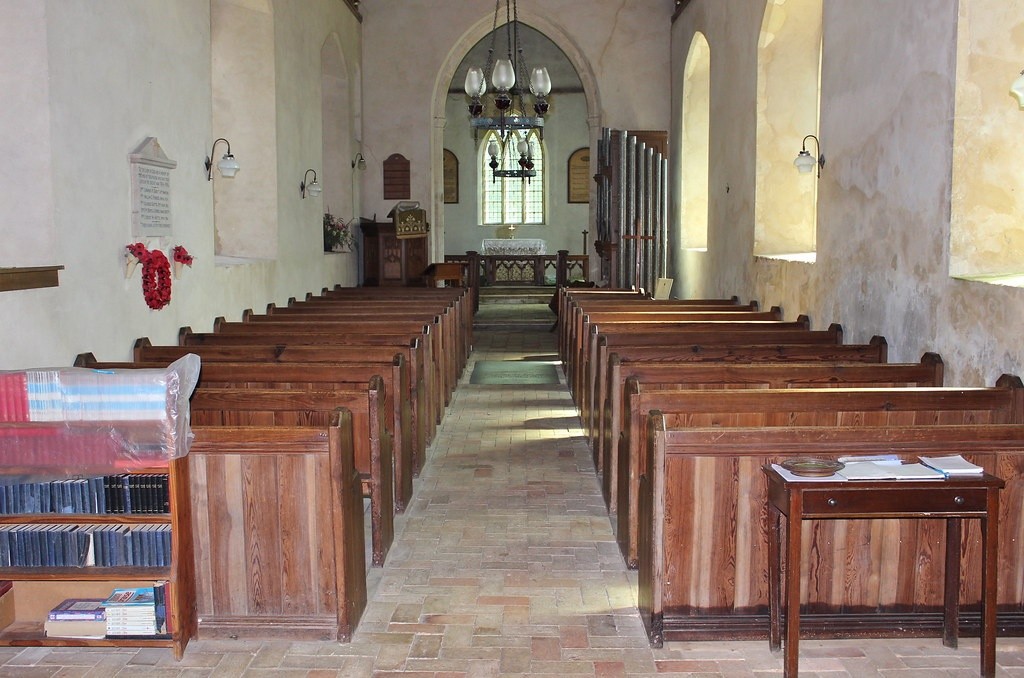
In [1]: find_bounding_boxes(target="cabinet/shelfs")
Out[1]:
[1,364,200,661]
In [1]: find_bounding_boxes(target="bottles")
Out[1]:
[509,225,515,239]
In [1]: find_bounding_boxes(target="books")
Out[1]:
[772,454,983,482]
[0,524,172,568]
[0,423,168,468]
[0,471,170,516]
[0,367,166,423]
[44,581,174,642]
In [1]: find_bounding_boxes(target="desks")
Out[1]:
[760,458,1006,678]
[482,238,547,282]
[420,262,469,287]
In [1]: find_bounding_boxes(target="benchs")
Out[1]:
[73,284,475,645]
[558,285,1024,650]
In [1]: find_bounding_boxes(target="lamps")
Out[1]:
[300,169,322,200]
[794,135,825,178]
[352,152,367,170]
[463,0,552,185]
[205,138,241,182]
